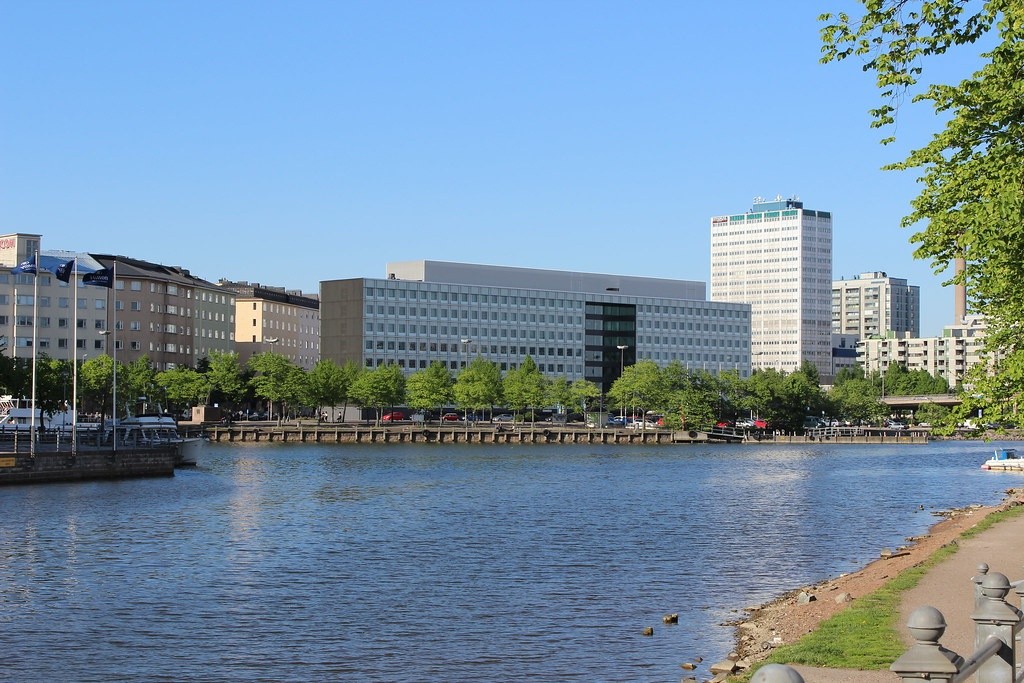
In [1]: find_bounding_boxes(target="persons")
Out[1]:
[321,411,342,422]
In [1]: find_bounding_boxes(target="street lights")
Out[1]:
[81,354,88,414]
[264,337,279,422]
[616,345,630,416]
[99,329,112,357]
[460,338,472,423]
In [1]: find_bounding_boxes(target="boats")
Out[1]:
[981,448,1024,471]
[0,394,102,439]
[103,396,204,465]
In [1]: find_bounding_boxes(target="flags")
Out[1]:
[83,265,115,290]
[10,254,37,277]
[47,259,76,284]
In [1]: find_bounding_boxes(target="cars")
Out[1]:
[442,413,459,422]
[409,410,434,420]
[606,411,911,430]
[382,410,405,421]
[545,411,584,424]
[234,410,294,421]
[461,412,483,421]
[492,413,513,422]
